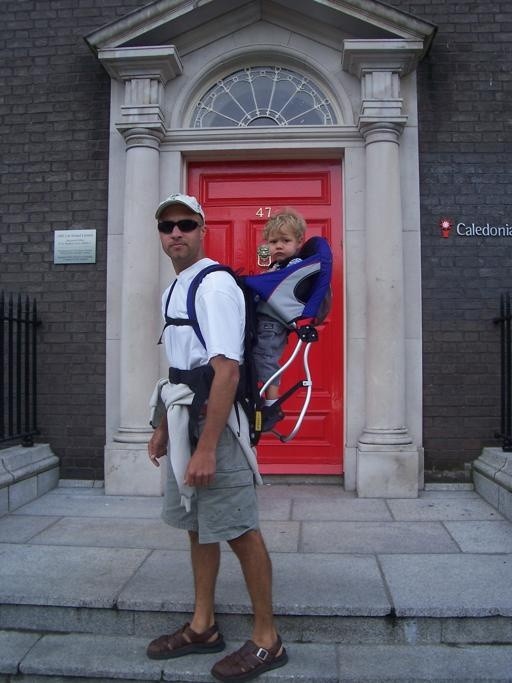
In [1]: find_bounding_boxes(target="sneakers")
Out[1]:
[250,403,286,433]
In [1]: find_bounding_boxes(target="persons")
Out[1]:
[243,206,305,433]
[147,191,289,679]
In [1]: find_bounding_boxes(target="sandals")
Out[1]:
[144,618,227,660]
[209,632,291,681]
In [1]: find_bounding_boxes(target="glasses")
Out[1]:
[156,217,204,233]
